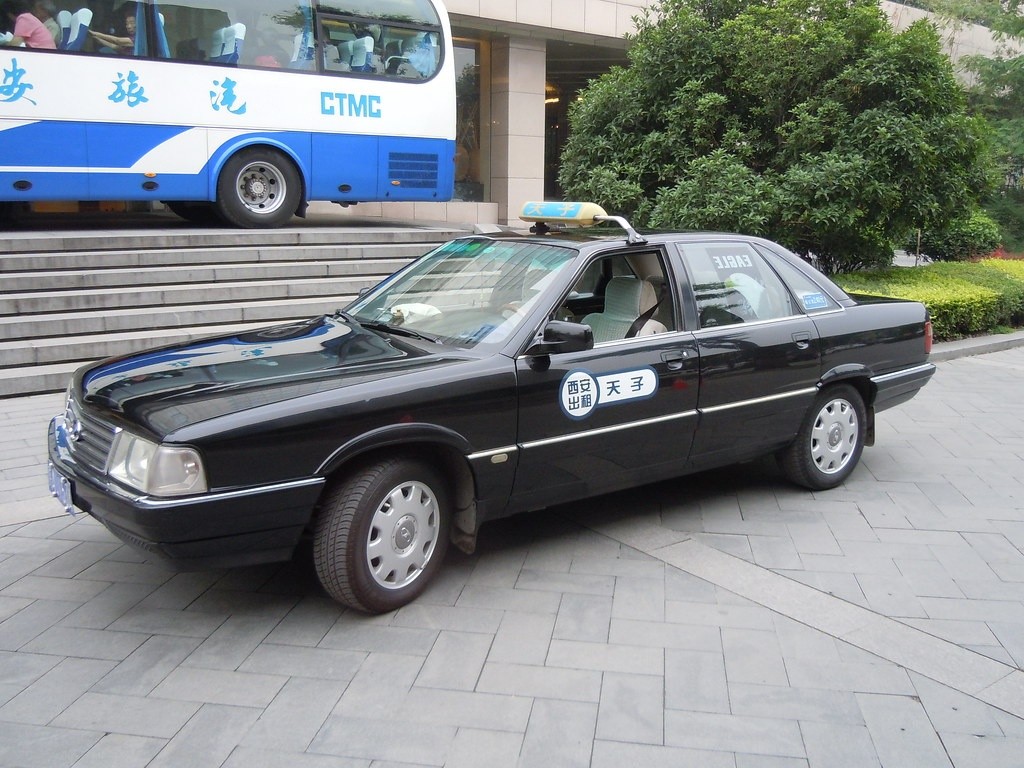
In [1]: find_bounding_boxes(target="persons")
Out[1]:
[88,11,138,56]
[383,42,402,75]
[4,0,58,50]
[246,25,290,68]
[321,24,340,72]
[33,0,62,49]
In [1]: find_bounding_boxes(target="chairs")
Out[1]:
[578,276,668,343]
[0,1,438,78]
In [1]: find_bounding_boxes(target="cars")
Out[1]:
[48,201,937,615]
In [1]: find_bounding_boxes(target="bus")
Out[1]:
[82,321,356,404]
[0,0,457,234]
